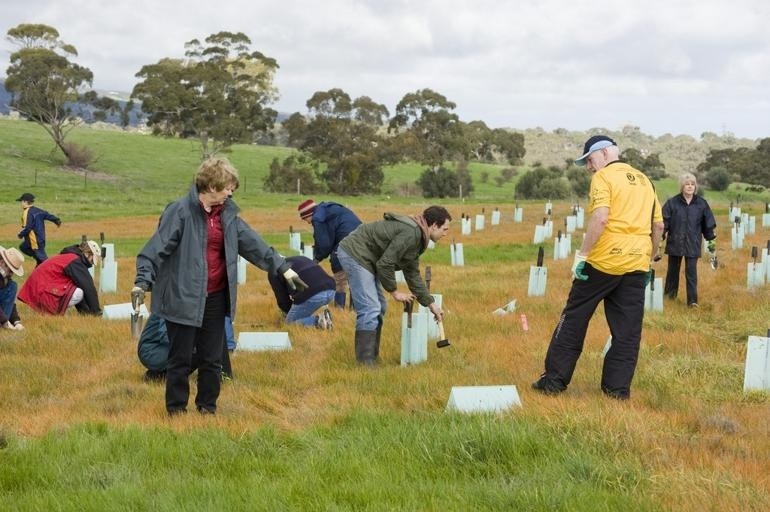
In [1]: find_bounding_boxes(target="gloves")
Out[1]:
[642,265,654,289]
[707,240,716,253]
[130,286,146,310]
[569,249,590,282]
[282,267,310,291]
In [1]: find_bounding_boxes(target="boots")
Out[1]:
[333,291,347,311]
[352,324,387,371]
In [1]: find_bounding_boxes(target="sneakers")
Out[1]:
[319,309,333,331]
[531,371,568,396]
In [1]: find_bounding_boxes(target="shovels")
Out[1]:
[709,242,718,271]
[130,295,143,336]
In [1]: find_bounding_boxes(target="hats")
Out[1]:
[0,244,25,277]
[16,192,35,202]
[679,171,698,195]
[574,134,618,167]
[298,199,317,219]
[87,239,102,266]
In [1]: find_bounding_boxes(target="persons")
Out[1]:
[17,241,103,319]
[268,255,335,332]
[337,205,452,364]
[299,200,362,311]
[0,245,25,330]
[138,309,235,381]
[131,158,309,415]
[661,173,716,307]
[531,135,666,398]
[15,193,61,267]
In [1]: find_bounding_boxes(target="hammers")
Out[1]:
[437,315,450,348]
[654,240,663,262]
